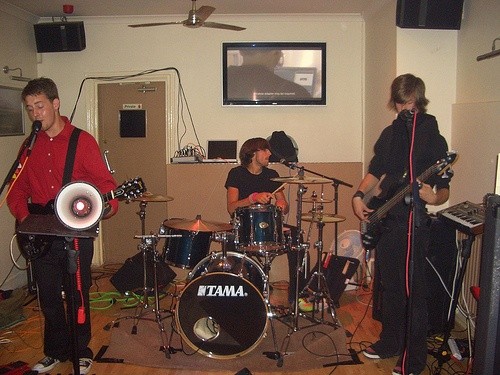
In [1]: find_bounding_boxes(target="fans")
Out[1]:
[128,0,247,31]
[329,229,365,291]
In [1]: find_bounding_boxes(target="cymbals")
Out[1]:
[269,175,333,185]
[296,197,332,203]
[296,212,346,223]
[163,217,234,233]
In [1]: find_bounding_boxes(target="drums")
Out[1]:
[162,227,213,271]
[234,203,283,252]
[222,227,293,256]
[174,250,270,360]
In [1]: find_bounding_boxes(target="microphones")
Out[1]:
[400,108,416,120]
[26,119,43,156]
[280,159,295,171]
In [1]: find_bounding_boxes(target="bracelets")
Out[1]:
[354,191,364,199]
[249,192,257,204]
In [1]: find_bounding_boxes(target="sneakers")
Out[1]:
[72,357,93,374]
[31,355,60,373]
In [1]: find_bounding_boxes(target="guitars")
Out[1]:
[14,175,148,262]
[359,150,458,250]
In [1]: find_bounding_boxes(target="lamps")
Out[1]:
[476,38,500,61]
[3,66,32,82]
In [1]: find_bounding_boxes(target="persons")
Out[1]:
[352,73,454,375]
[227,49,311,98]
[7,78,118,375]
[225,138,312,312]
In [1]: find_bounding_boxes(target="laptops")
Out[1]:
[202,140,237,162]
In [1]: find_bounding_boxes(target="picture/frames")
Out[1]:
[0,85,25,137]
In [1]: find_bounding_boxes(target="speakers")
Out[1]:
[34,21,86,54]
[110,248,177,298]
[395,0,464,30]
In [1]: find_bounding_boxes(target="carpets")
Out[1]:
[92,282,365,371]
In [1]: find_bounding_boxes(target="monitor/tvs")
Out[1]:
[222,42,326,105]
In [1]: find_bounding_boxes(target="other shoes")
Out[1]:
[392,365,409,375]
[363,347,380,358]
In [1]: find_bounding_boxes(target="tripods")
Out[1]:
[269,182,352,367]
[104,203,193,360]
